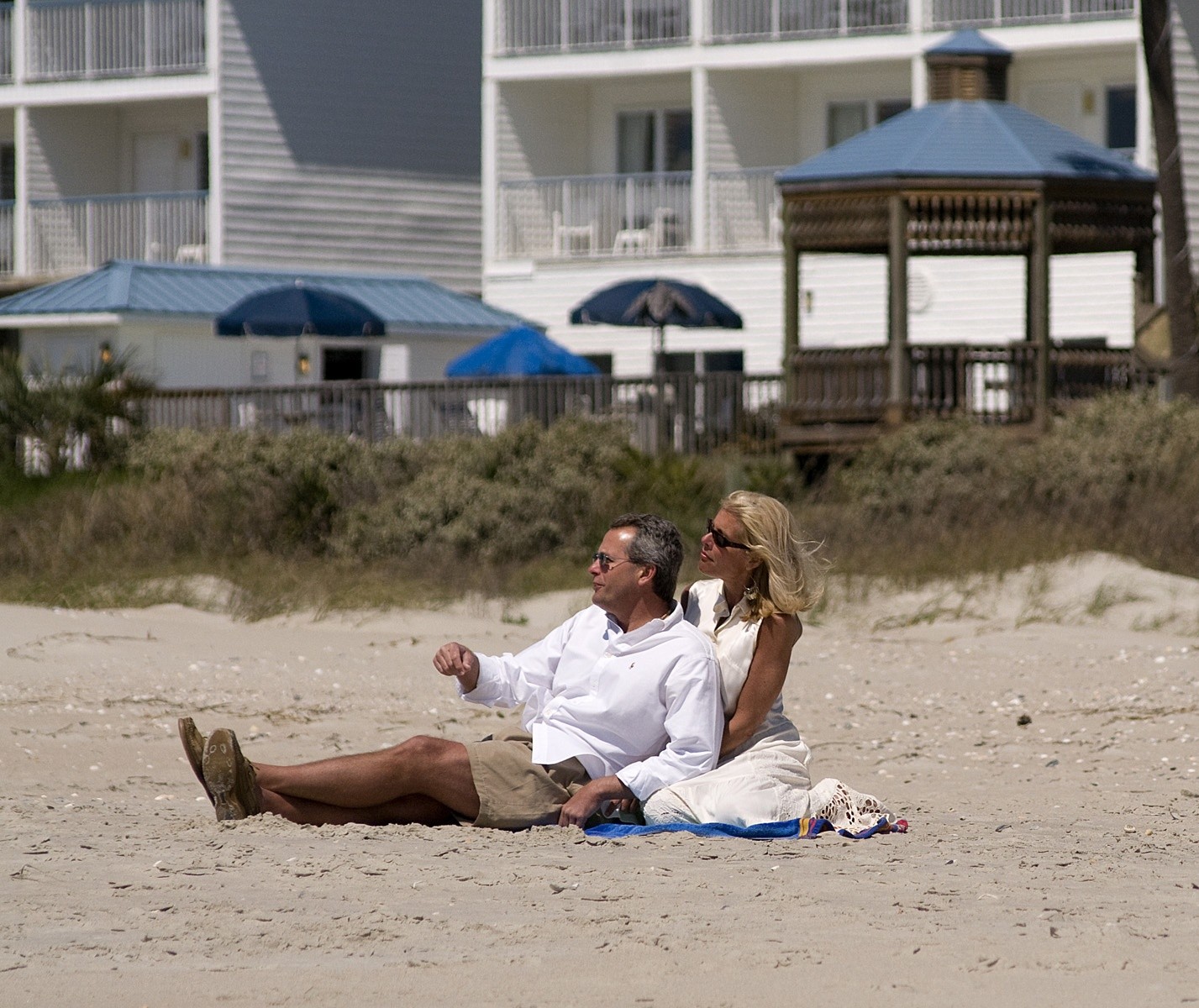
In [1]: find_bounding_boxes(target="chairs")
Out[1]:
[552,212,600,256]
[615,206,678,253]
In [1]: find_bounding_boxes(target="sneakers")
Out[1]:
[178,717,215,805]
[201,729,265,821]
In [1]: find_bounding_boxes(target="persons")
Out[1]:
[601,491,897,833]
[178,512,726,830]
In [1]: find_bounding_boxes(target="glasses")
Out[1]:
[592,552,639,567]
[705,518,753,551]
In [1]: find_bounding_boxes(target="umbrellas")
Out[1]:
[444,327,591,377]
[214,282,387,382]
[569,279,742,370]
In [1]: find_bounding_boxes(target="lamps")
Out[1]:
[98,341,110,363]
[297,354,310,376]
